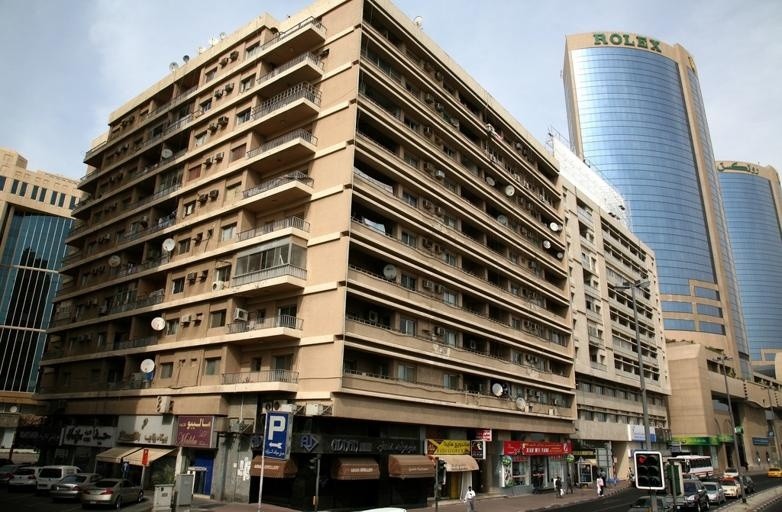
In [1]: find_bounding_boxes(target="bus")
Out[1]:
[676,455,714,479]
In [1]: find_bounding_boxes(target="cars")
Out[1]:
[767,467,782,478]
[628,474,710,511]
[0,463,144,509]
[703,468,753,505]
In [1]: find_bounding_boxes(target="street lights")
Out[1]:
[610,279,654,453]
[761,385,780,463]
[713,354,746,502]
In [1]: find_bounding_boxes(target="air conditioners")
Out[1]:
[139,216,149,224]
[424,200,434,208]
[233,307,248,322]
[529,261,537,268]
[435,169,446,179]
[527,231,535,238]
[436,327,445,336]
[219,57,228,64]
[486,123,496,132]
[104,233,110,240]
[181,314,190,322]
[425,127,434,135]
[528,389,534,396]
[215,152,224,159]
[426,93,435,103]
[489,154,498,162]
[512,174,521,181]
[96,305,107,314]
[209,189,220,198]
[207,123,217,130]
[203,157,213,164]
[436,207,446,215]
[218,116,228,124]
[438,285,446,294]
[186,272,194,279]
[527,354,534,361]
[212,281,223,291]
[435,103,443,110]
[224,82,234,90]
[230,51,238,59]
[534,323,541,330]
[425,162,434,171]
[536,391,543,397]
[197,194,207,201]
[424,280,434,289]
[213,89,222,96]
[523,181,529,188]
[525,320,534,327]
[437,246,444,253]
[259,396,291,415]
[424,237,433,248]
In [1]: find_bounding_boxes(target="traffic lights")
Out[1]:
[637,454,662,486]
[437,460,445,485]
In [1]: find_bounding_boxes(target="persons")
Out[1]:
[600,469,607,488]
[596,473,604,496]
[626,466,633,486]
[555,475,562,497]
[463,486,475,512]
[565,473,573,494]
